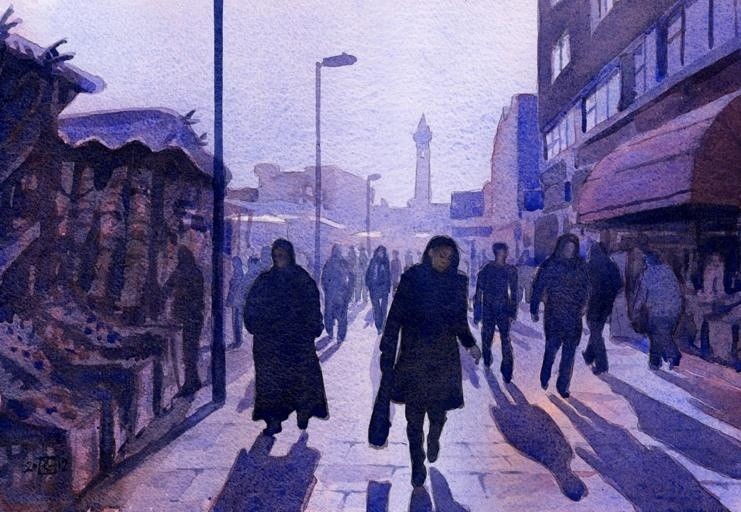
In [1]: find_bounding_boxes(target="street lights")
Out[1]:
[365,173,380,257]
[314,51,357,284]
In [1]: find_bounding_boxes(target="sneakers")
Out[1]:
[426,435,440,462]
[410,464,427,486]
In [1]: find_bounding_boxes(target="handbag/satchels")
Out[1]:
[368,387,392,447]
